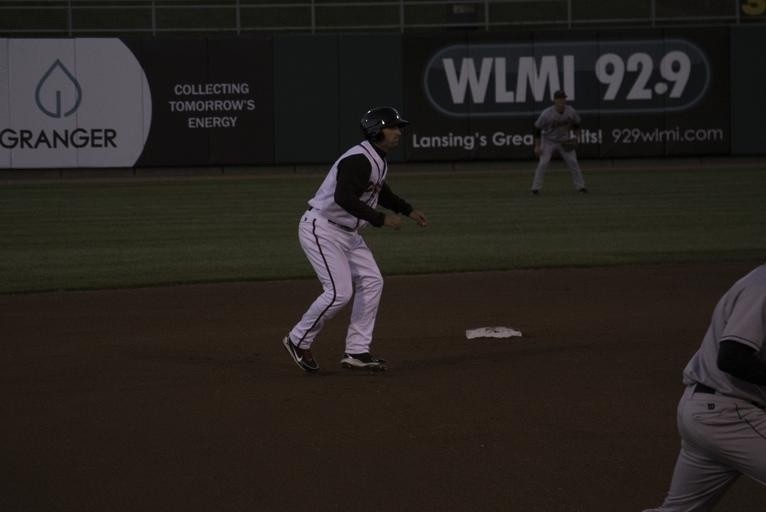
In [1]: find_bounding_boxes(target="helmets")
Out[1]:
[361,107,412,138]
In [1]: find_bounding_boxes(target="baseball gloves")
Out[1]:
[560,137,579,151]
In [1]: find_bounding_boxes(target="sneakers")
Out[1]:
[578,188,587,193]
[532,189,539,195]
[341,353,389,372]
[283,335,319,373]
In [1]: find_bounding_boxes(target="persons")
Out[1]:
[652,259,766,512]
[282,107,430,375]
[530,89,592,198]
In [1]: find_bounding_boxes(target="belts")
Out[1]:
[694,383,718,394]
[308,205,354,232]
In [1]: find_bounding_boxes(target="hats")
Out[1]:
[554,90,567,98]
